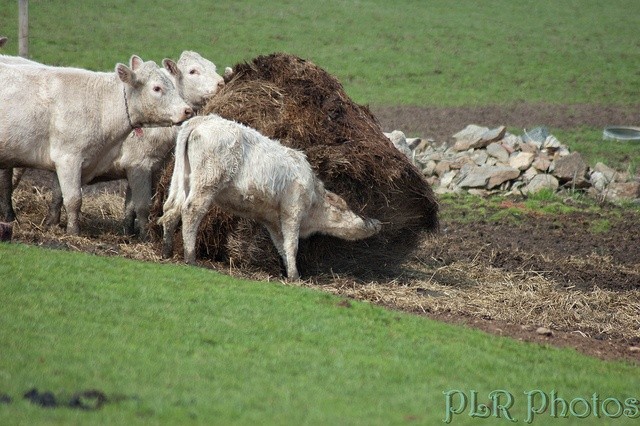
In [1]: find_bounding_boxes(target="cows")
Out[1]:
[156,113,384,282]
[0,51,227,242]
[1,53,195,242]
